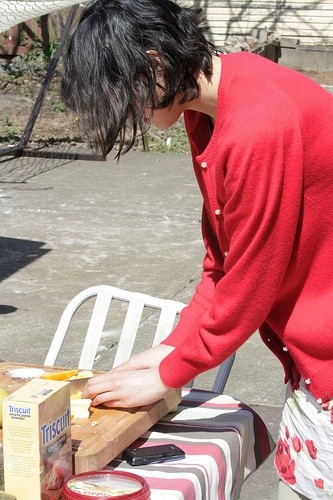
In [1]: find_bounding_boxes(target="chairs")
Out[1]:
[41,284,236,393]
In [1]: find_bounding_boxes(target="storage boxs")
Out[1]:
[2,379,72,500]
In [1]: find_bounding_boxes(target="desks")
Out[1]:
[0,365,277,500]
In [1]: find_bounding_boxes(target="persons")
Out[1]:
[59,0,333,500]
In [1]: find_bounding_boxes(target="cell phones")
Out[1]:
[124,443,185,467]
